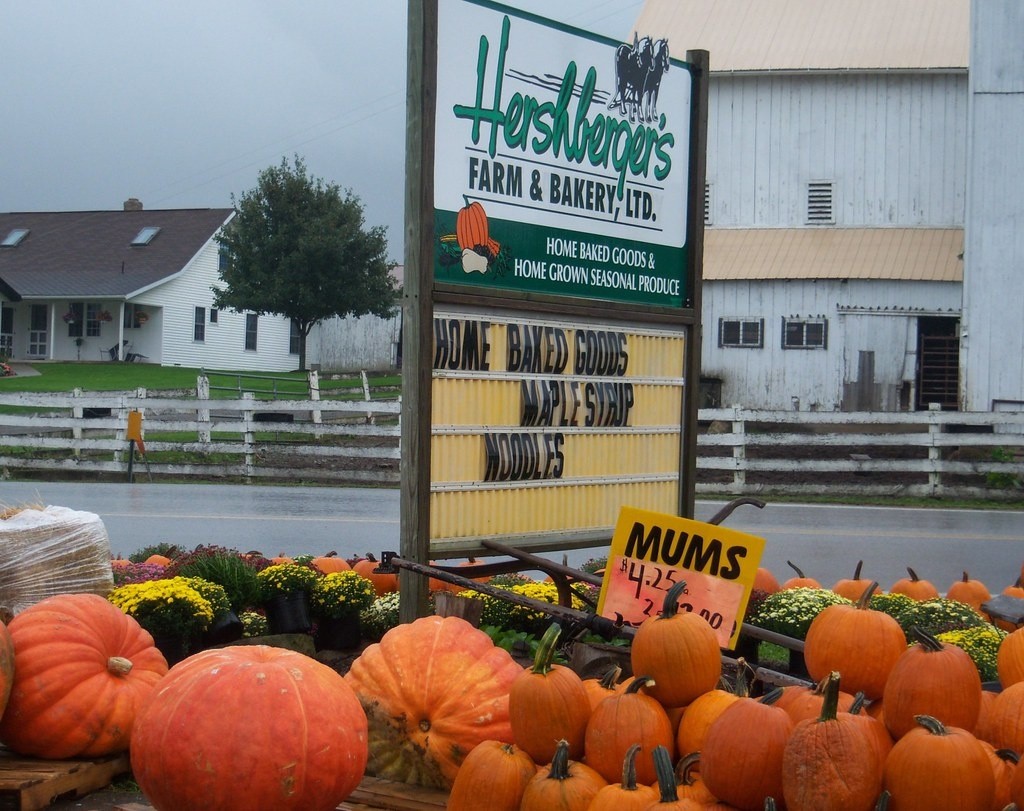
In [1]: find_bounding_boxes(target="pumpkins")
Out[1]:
[0,546,1024,811]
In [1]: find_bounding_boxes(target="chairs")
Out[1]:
[99,339,150,363]
